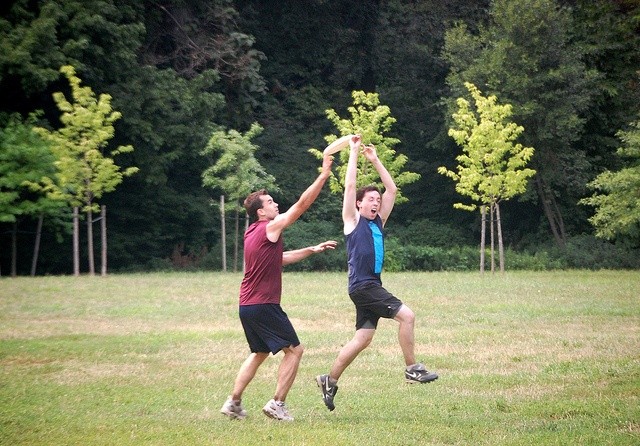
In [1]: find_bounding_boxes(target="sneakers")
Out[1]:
[262,399,295,422]
[220,396,247,421]
[405,363,438,384]
[314,374,339,412]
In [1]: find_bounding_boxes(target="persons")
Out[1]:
[224,155,339,423]
[313,137,437,410]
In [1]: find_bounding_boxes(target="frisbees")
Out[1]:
[322,134,354,156]
